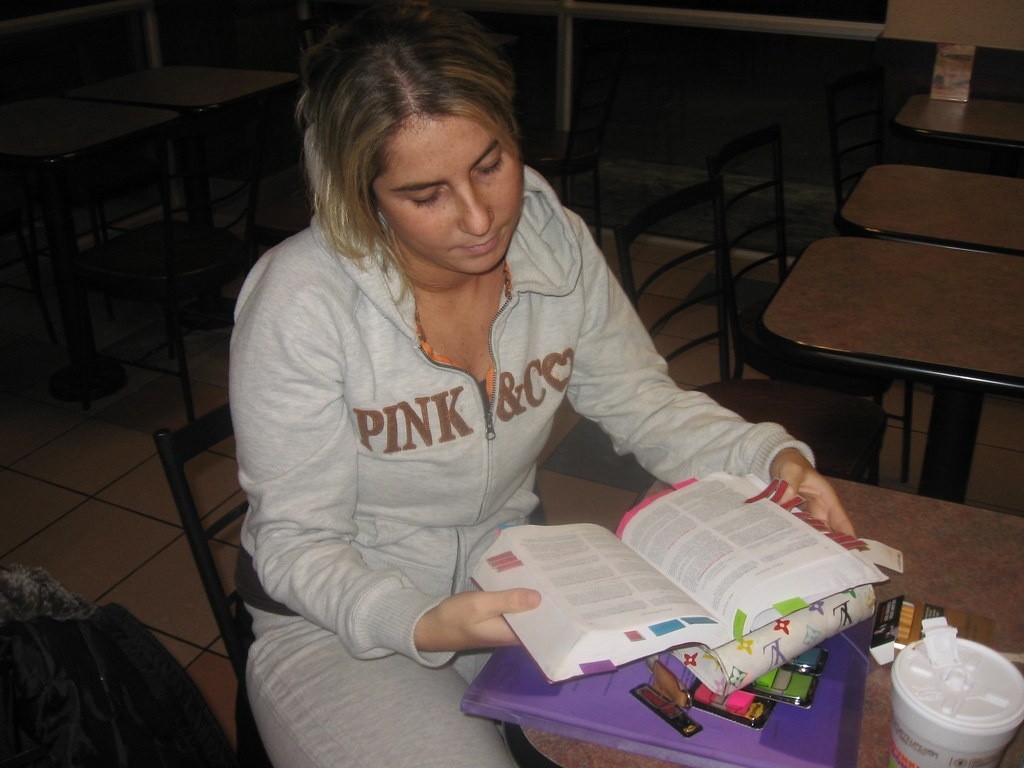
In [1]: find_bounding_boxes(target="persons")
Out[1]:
[229,0,857,768]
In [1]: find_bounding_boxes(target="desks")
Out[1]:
[835,163,1024,258]
[889,94,1024,180]
[501,467,1024,768]
[756,236,1024,504]
[0,97,182,402]
[64,63,300,330]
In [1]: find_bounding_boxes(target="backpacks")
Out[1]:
[0,601,238,768]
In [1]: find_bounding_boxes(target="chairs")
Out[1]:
[79,17,923,768]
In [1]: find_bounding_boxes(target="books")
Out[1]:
[472,472,889,684]
